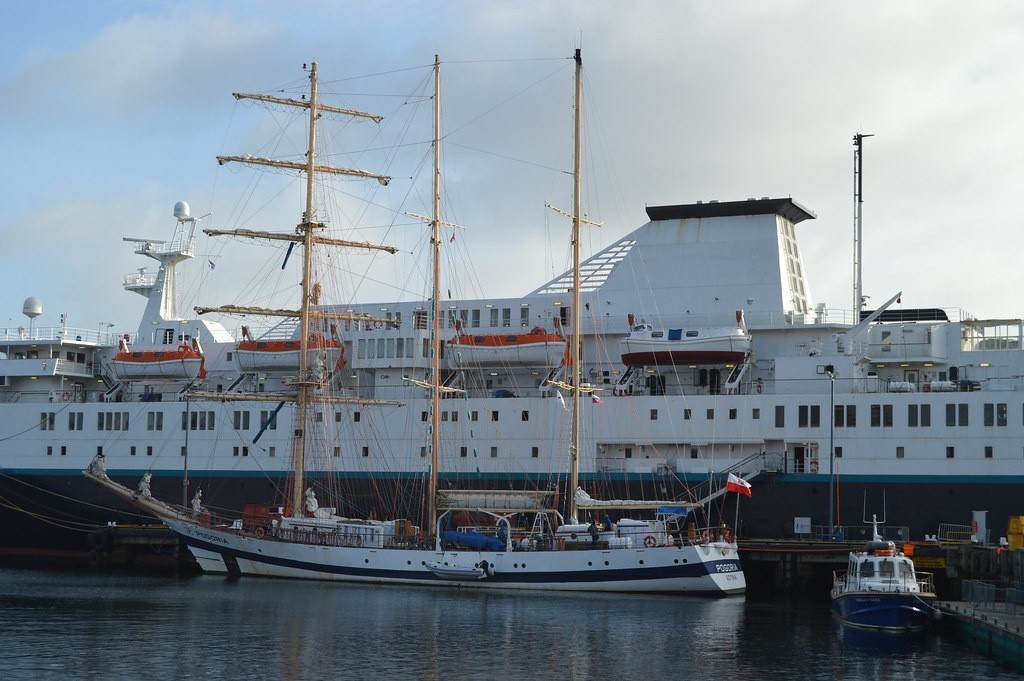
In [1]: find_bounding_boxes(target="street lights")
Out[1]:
[825,369,834,542]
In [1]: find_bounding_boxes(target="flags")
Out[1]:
[593,394,601,404]
[556,392,569,413]
[726,472,752,498]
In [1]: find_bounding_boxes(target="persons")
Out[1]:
[604,514,610,530]
[498,524,547,551]
[588,518,600,541]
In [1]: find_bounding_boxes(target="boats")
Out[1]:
[0,195,1022,550]
[828,513,943,642]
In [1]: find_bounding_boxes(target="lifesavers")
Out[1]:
[810,460,818,472]
[874,550,893,556]
[643,536,657,548]
[757,385,762,394]
[62,392,70,402]
[374,321,383,329]
[702,531,707,544]
[255,527,264,538]
[724,529,732,543]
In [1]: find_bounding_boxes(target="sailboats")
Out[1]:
[75,40,754,597]
